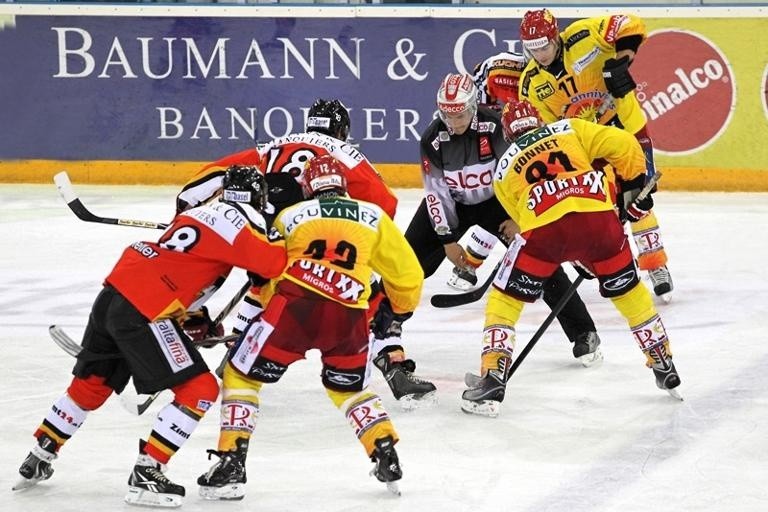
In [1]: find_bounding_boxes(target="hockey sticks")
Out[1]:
[53,171,171,230]
[119,280,254,415]
[466,172,662,387]
[430,93,615,308]
[49,324,241,357]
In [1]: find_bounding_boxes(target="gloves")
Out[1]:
[370,297,413,340]
[601,55,636,98]
[617,173,653,224]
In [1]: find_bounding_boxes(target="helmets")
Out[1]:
[222,99,350,213]
[436,8,560,147]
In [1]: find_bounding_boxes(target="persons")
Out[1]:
[195,152,427,489]
[172,97,438,403]
[19,163,288,498]
[367,6,682,416]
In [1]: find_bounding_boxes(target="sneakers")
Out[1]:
[384,368,437,401]
[18,443,57,481]
[573,331,601,359]
[653,359,680,391]
[197,452,246,489]
[648,264,673,297]
[127,465,185,500]
[461,369,508,404]
[369,452,402,483]
[452,257,478,288]
